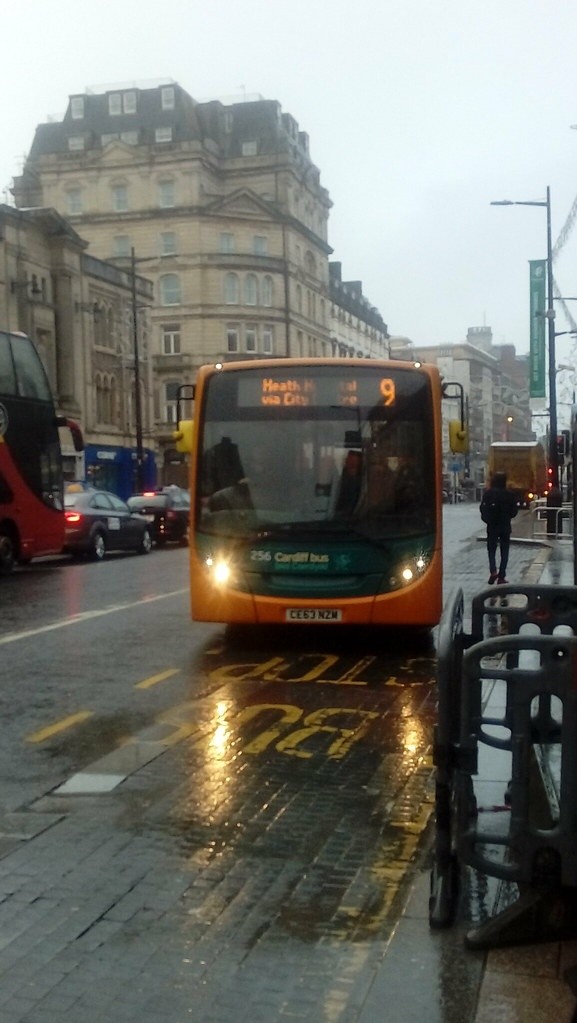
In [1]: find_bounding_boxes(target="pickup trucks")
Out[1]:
[488,441,546,509]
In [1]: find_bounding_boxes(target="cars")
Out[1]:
[126,483,191,549]
[65,484,154,560]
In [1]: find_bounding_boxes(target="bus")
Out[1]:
[174,357,468,645]
[0,330,84,576]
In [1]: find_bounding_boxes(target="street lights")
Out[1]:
[491,186,576,538]
[125,246,152,495]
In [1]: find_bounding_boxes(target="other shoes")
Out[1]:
[498,578,508,584]
[488,573,497,584]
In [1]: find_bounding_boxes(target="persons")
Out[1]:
[480,471,520,584]
[234,441,279,484]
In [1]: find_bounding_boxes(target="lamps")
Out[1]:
[9,272,45,295]
